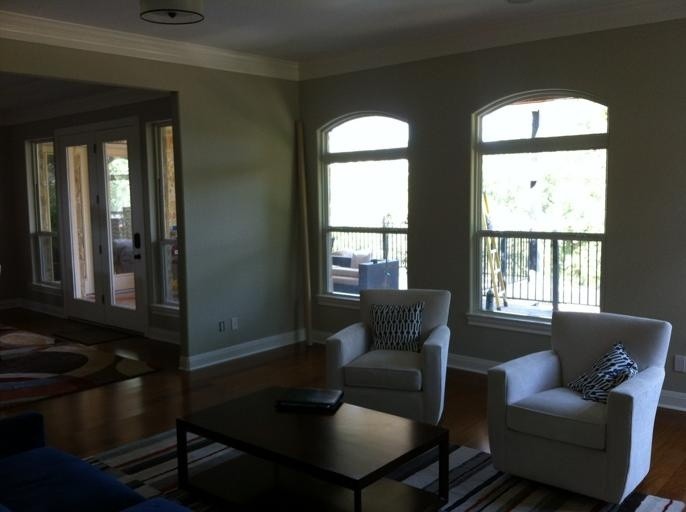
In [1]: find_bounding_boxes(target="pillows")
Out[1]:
[175,383,451,512]
[370,300,427,352]
[352,252,372,269]
[565,341,638,402]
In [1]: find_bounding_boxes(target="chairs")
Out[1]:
[487,310,674,506]
[323,288,453,426]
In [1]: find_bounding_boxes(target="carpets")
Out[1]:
[80,402,685,512]
[0,319,160,410]
[53,322,139,345]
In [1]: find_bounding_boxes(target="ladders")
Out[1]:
[480,190,507,311]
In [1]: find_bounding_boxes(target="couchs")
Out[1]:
[0,411,193,512]
[330,252,399,294]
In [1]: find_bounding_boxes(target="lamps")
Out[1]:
[140,1,204,25]
